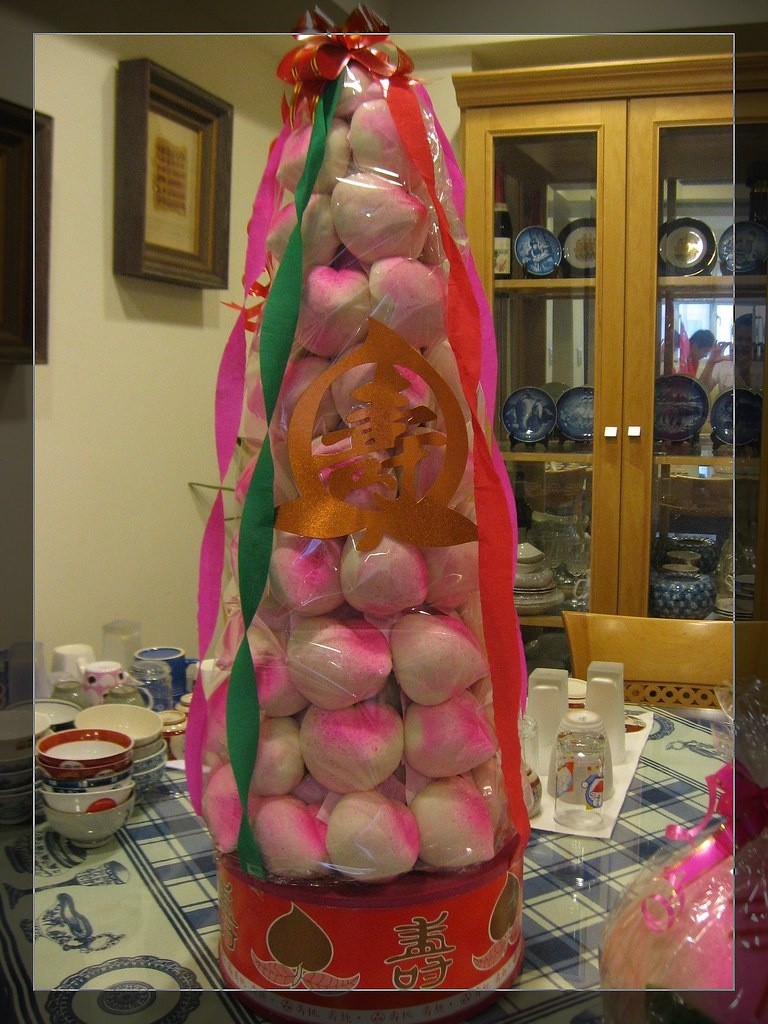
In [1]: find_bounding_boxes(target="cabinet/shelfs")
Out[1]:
[444,53,768,688]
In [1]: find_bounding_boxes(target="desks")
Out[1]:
[0,689,768,1024]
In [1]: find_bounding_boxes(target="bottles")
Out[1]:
[492,157,513,280]
[528,191,542,226]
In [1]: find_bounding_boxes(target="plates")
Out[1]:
[513,581,565,615]
[654,375,710,442]
[514,225,562,275]
[714,597,754,621]
[555,386,593,441]
[501,387,557,443]
[658,217,717,276]
[717,221,768,272]
[558,218,596,276]
[710,388,763,445]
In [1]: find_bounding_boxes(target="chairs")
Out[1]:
[556,606,768,721]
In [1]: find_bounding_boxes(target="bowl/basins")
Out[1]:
[0,698,169,849]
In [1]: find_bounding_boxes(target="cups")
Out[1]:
[0,619,203,763]
[520,661,627,829]
[725,574,754,612]
[573,570,590,612]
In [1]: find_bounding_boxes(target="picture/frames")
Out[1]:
[114,57,236,296]
